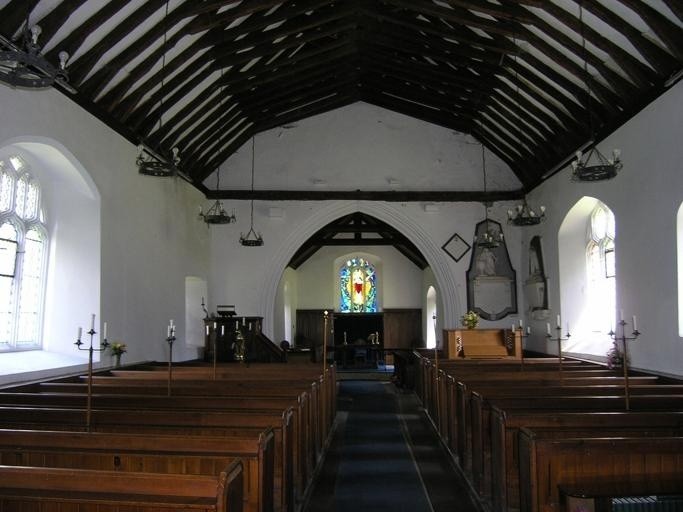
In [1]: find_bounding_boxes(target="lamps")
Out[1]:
[472,111,503,248]
[238,135,264,246]
[441,232,471,262]
[136,0,181,177]
[507,1,546,226]
[569,0,623,181]
[0,0,79,96]
[197,69,236,228]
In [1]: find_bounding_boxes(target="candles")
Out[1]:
[222,326,224,334]
[512,324,515,332]
[243,317,245,326]
[206,326,209,335]
[168,319,176,337]
[528,327,530,333]
[520,320,522,326]
[249,323,251,330]
[236,321,238,329]
[546,315,570,333]
[609,309,637,331]
[214,323,216,328]
[77,314,107,340]
